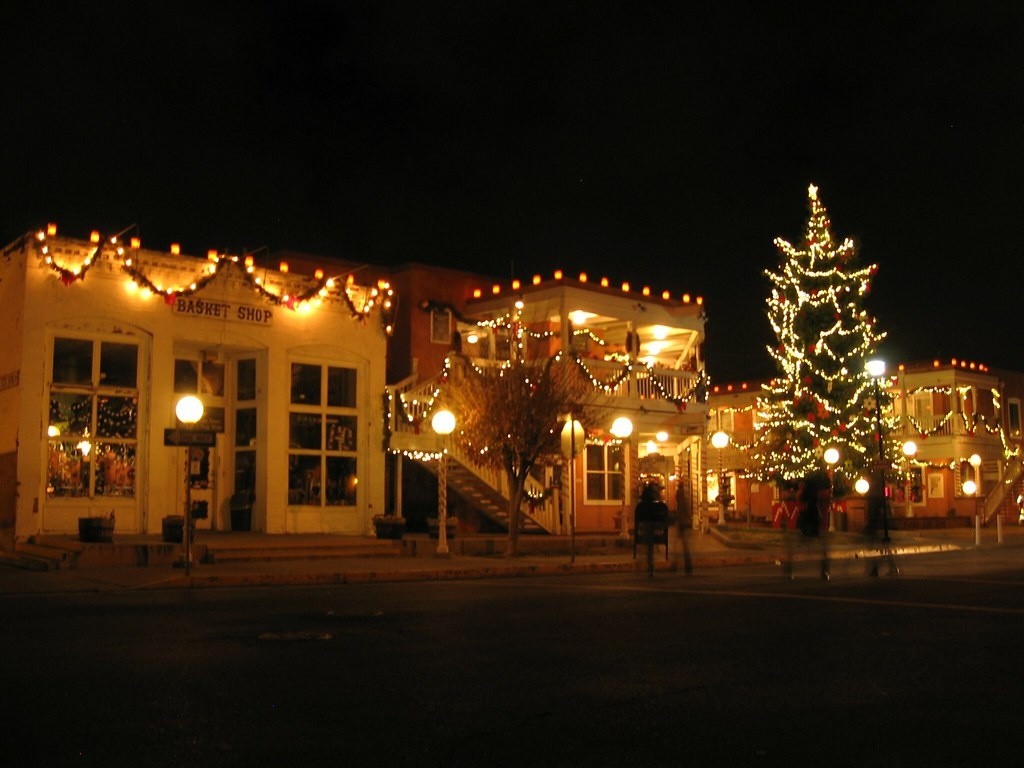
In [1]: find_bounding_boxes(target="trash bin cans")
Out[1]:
[230,494,253,532]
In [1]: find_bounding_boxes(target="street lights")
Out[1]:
[969,453,982,544]
[865,358,890,541]
[903,441,917,519]
[175,396,204,576]
[432,410,456,553]
[612,418,633,539]
[824,448,839,532]
[712,431,728,525]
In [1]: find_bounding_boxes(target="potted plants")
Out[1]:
[370,513,406,540]
[428,515,458,540]
